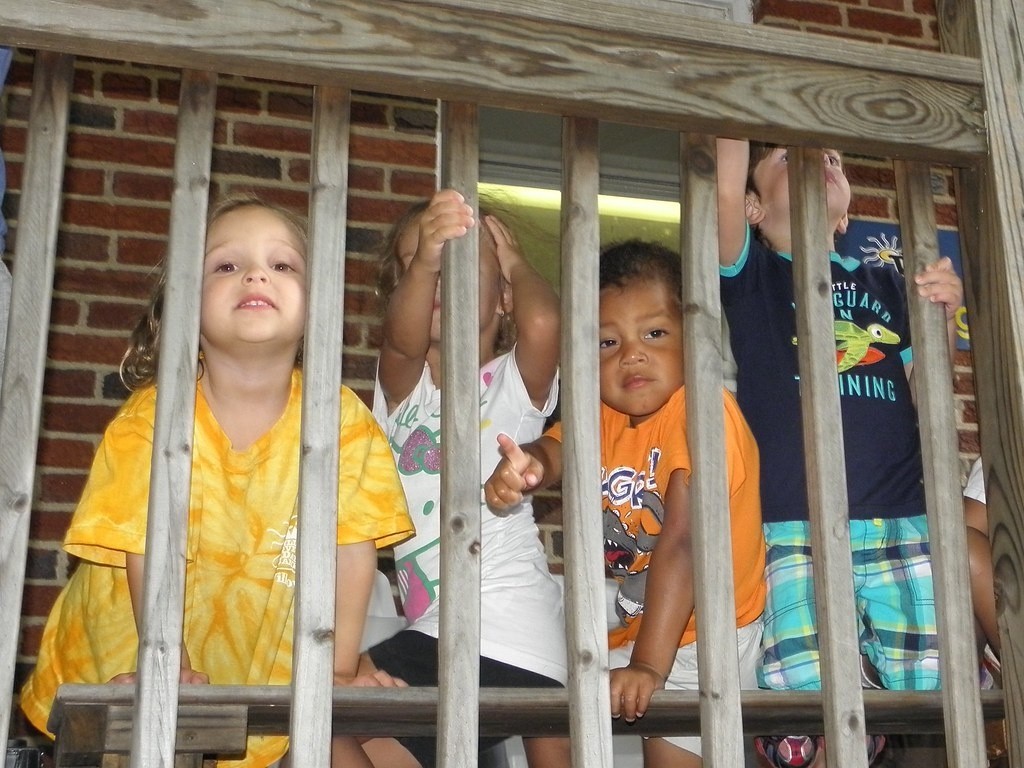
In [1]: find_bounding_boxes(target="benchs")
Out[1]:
[47,681,1006,768]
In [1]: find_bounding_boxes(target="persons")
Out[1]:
[368,126,1001,768]
[16,188,415,768]
